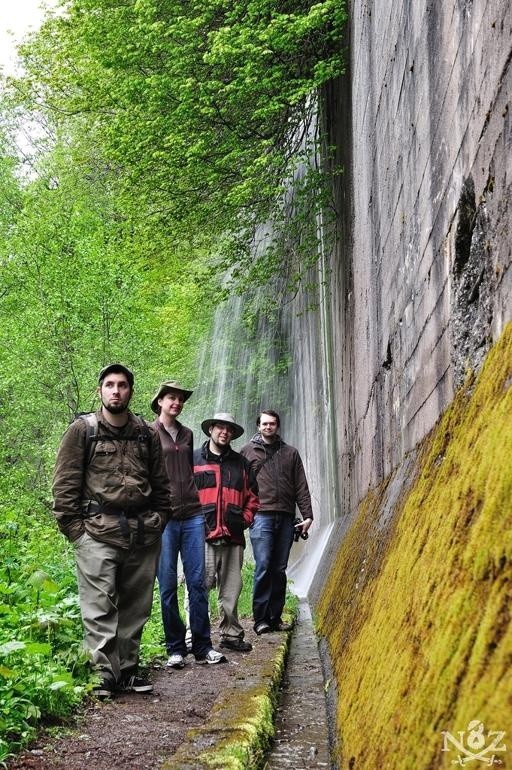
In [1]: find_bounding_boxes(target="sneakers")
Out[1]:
[166,653,184,669]
[185,620,294,651]
[195,649,227,665]
[117,675,154,693]
[91,678,115,696]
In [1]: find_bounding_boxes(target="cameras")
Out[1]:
[292,518,308,542]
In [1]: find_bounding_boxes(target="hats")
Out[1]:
[151,380,193,415]
[202,413,244,440]
[98,363,134,385]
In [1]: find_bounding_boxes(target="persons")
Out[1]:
[182,413,260,655]
[49,362,172,702]
[147,378,225,669]
[240,409,314,635]
